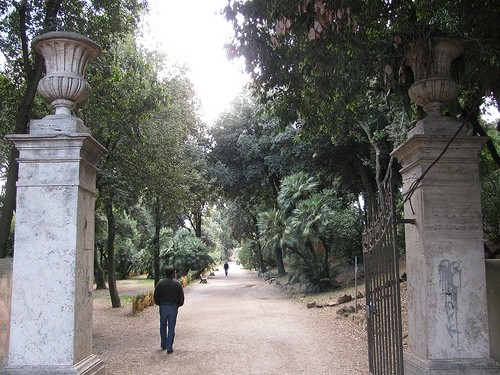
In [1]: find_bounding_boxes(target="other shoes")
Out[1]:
[161,345,166,350]
[167,349,174,353]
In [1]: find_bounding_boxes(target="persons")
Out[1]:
[153,266,184,355]
[224,261,230,275]
